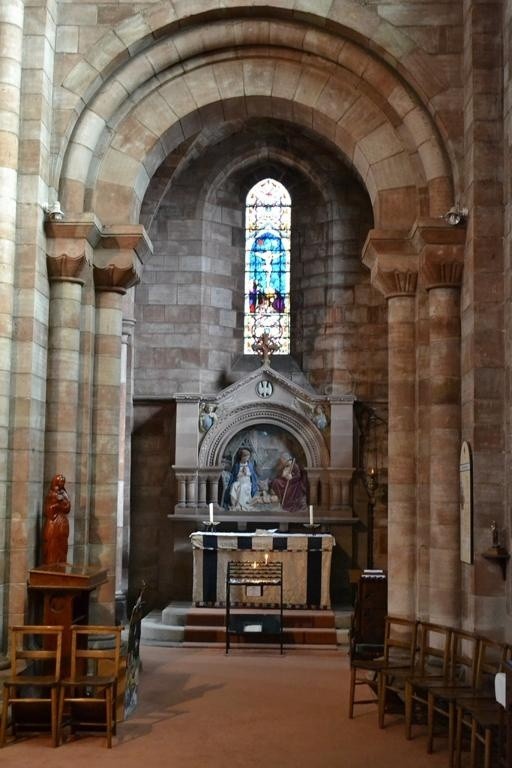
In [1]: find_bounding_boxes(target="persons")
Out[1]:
[226,450,256,512]
[312,405,328,429]
[200,403,218,432]
[270,452,308,512]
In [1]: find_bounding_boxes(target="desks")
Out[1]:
[190,530,336,610]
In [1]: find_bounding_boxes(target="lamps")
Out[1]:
[43,201,64,220]
[445,206,469,226]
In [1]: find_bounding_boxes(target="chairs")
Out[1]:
[57,624,125,748]
[0,625,64,749]
[349,615,512,768]
